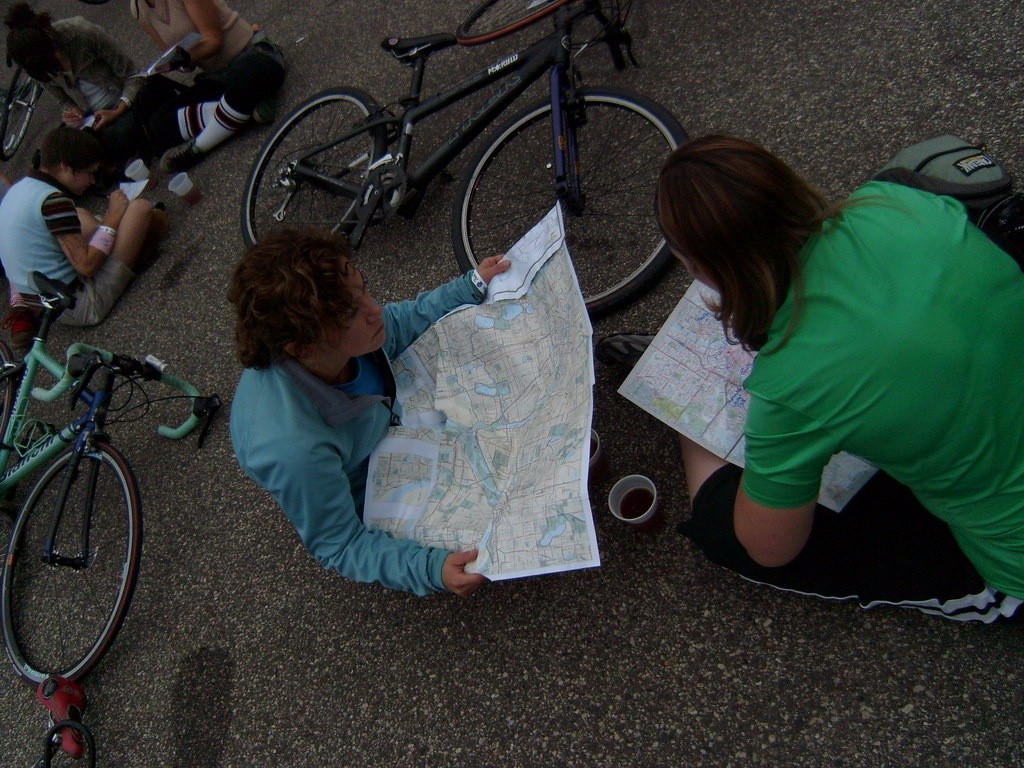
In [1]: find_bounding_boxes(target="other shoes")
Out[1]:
[92,165,115,195]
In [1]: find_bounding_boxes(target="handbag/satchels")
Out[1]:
[870,135,1013,212]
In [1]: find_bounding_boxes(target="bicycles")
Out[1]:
[0,269,222,689]
[0,66,43,161]
[243,0,689,324]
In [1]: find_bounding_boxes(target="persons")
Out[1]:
[226,221,512,598]
[0,0,287,349]
[595,134,1023,628]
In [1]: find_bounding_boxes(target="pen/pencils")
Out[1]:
[64,111,70,113]
[95,195,110,198]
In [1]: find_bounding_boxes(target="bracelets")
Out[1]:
[88,230,115,256]
[116,96,131,108]
[471,267,488,296]
[98,225,116,236]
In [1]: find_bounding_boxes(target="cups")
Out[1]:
[124,159,157,190]
[588,428,609,483]
[607,475,663,534]
[168,172,200,205]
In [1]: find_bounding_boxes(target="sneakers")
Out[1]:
[2,307,37,348]
[594,333,658,374]
[253,96,276,124]
[160,137,208,174]
